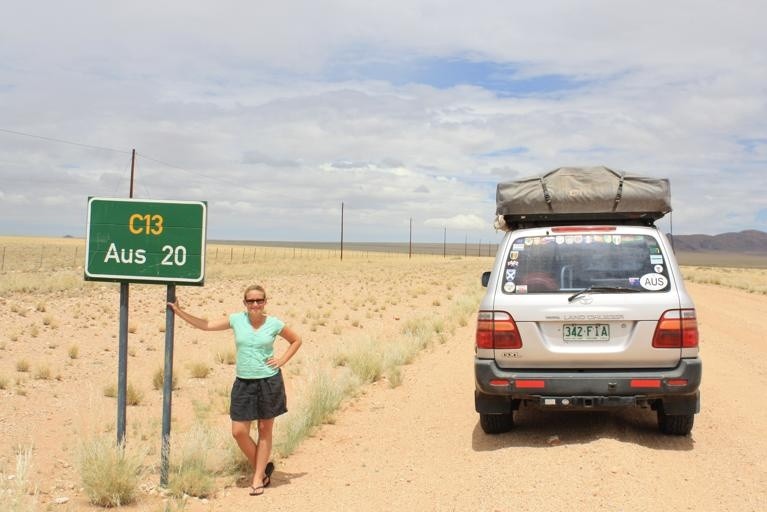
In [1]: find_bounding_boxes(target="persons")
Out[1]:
[167,284,302,495]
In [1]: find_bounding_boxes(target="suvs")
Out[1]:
[469,163,704,439]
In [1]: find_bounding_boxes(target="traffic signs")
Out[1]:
[84,196,206,286]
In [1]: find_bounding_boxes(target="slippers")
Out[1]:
[250,462,274,494]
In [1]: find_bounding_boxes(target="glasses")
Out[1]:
[245,298,264,304]
